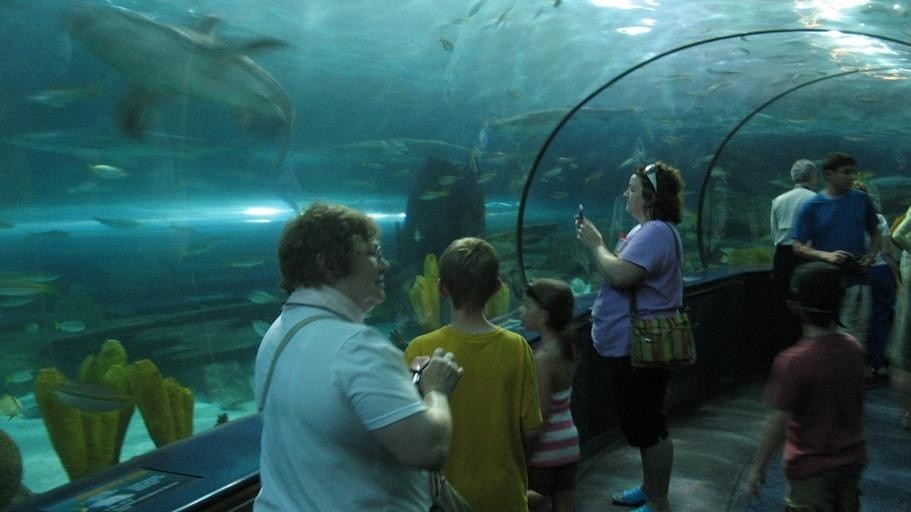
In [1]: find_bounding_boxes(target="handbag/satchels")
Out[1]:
[429,469,470,511]
[629,223,698,369]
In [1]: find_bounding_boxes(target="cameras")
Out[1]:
[841,254,863,272]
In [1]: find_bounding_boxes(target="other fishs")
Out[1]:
[330,2,909,248]
[0,0,287,421]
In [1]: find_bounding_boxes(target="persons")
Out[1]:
[736,260,871,512]
[885,204,911,428]
[768,155,824,355]
[251,203,466,512]
[573,157,691,512]
[404,237,543,512]
[851,181,901,379]
[787,147,883,391]
[510,276,584,512]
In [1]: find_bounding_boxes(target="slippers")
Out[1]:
[630,504,652,512]
[612,484,647,504]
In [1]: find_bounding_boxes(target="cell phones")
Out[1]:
[579,204,584,224]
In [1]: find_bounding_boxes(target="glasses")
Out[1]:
[367,244,382,261]
[643,163,658,190]
